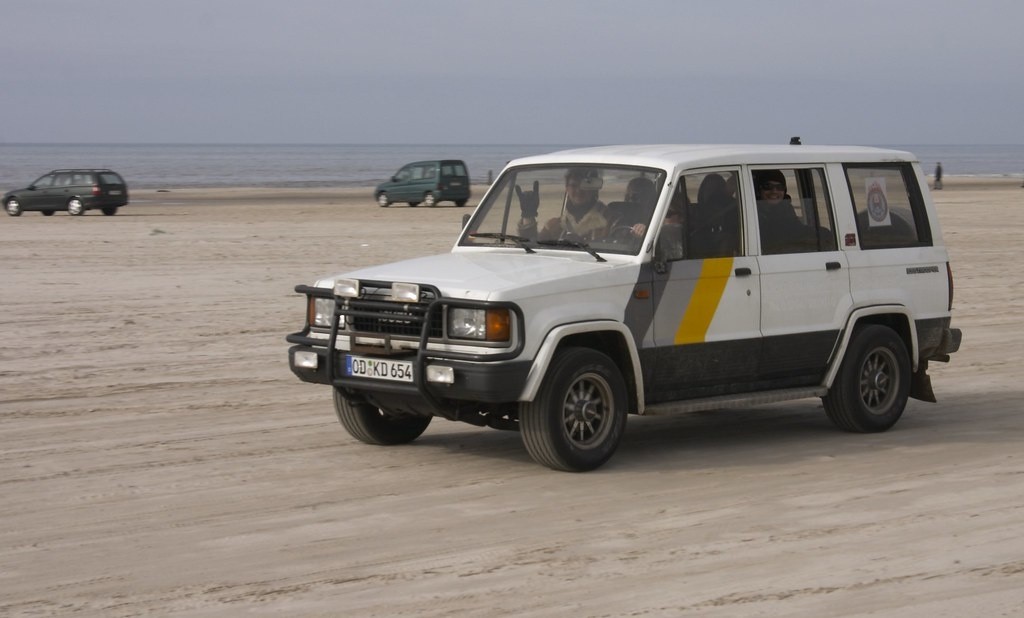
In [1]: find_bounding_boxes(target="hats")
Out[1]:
[756,169,786,183]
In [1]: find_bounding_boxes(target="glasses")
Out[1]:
[665,209,678,218]
[761,182,786,191]
[625,193,640,201]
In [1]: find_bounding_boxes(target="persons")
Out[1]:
[624,169,803,258]
[516,166,624,243]
[934,162,942,189]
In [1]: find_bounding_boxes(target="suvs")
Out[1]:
[0,167,129,217]
[286,137,961,473]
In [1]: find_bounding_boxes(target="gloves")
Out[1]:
[515,181,540,217]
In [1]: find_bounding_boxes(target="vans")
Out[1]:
[374,159,473,208]
